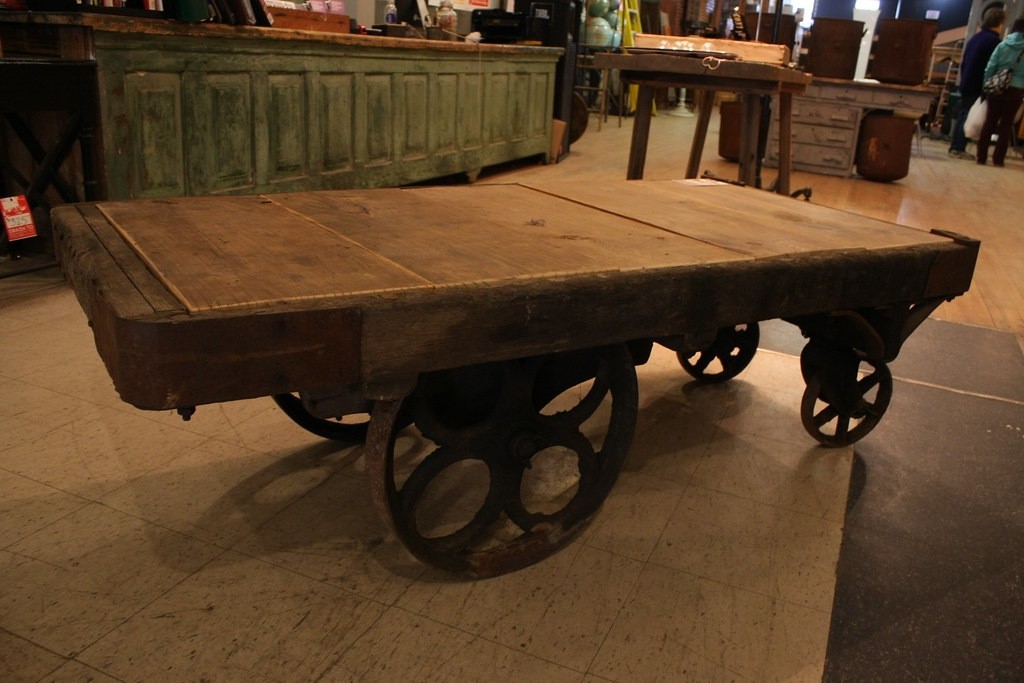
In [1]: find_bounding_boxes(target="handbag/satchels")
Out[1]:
[963,96,988,142]
[983,48,1024,97]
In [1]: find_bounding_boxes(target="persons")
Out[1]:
[947,7,1006,154]
[792,7,805,63]
[975,18,1023,166]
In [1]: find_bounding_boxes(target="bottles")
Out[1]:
[384,0,397,24]
[438,0,458,41]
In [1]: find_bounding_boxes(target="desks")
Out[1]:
[594,52,814,197]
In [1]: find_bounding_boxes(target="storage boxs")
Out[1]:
[267,6,351,35]
[0,193,37,242]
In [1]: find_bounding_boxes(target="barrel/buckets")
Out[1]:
[857,112,914,182]
[873,18,940,86]
[804,16,865,80]
[745,12,798,62]
[718,101,742,162]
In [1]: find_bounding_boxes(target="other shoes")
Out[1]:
[993,161,1004,166]
[977,159,985,164]
[948,149,975,160]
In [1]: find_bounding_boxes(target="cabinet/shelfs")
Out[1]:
[761,76,941,180]
[0,7,567,204]
